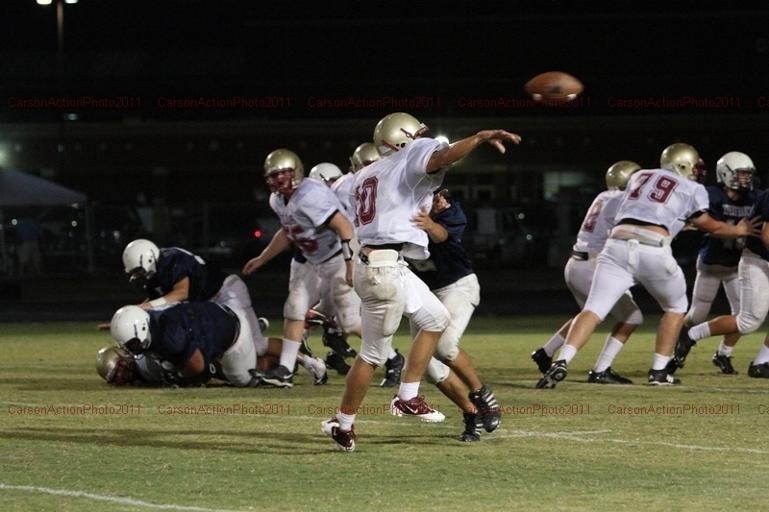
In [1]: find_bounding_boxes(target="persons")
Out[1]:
[349,144,502,443]
[531,143,768,391]
[320,110,524,452]
[0,210,97,323]
[97,139,407,388]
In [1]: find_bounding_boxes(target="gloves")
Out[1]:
[159,368,183,387]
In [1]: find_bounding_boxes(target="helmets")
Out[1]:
[262,148,304,195]
[605,160,642,189]
[309,162,343,184]
[123,239,160,286]
[660,142,711,183]
[110,304,153,355]
[715,152,755,192]
[374,112,429,158]
[350,141,379,171]
[95,346,138,384]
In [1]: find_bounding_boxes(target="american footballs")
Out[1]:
[523,71,586,107]
